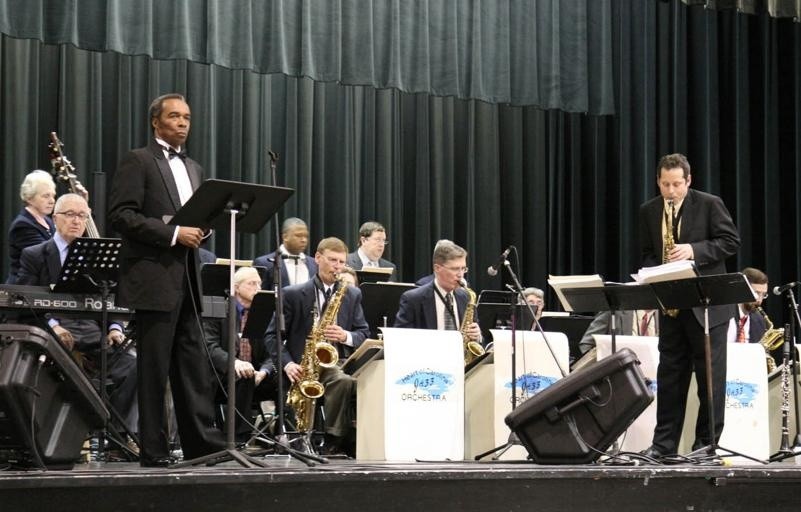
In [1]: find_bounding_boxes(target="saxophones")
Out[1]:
[659,200,681,317]
[286,311,325,432]
[754,303,784,375]
[315,272,348,368]
[456,279,486,367]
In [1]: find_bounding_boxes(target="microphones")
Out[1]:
[486,246,512,278]
[774,282,795,298]
[281,252,300,264]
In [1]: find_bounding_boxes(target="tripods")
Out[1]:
[561,280,662,467]
[205,150,329,468]
[166,179,296,470]
[52,238,140,464]
[763,289,801,462]
[649,273,768,466]
[474,260,524,462]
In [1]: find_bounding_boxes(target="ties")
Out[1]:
[442,293,458,331]
[239,310,252,362]
[737,316,749,344]
[320,290,332,318]
[639,315,649,337]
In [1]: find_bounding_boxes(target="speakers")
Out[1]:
[506,347,656,468]
[1,324,113,473]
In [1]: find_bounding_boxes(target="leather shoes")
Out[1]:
[222,442,248,452]
[139,455,178,468]
[629,446,663,464]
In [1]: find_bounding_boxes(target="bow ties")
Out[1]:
[161,143,188,162]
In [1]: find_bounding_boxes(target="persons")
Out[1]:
[628,154,741,459]
[516,287,544,331]
[579,308,659,355]
[348,222,396,283]
[110,93,248,468]
[253,217,318,291]
[416,239,454,286]
[393,246,485,347]
[738,268,770,343]
[6,170,56,286]
[15,193,140,455]
[264,237,371,438]
[202,268,274,423]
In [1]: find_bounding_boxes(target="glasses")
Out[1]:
[362,236,388,247]
[53,211,91,222]
[758,292,768,300]
[439,262,468,277]
